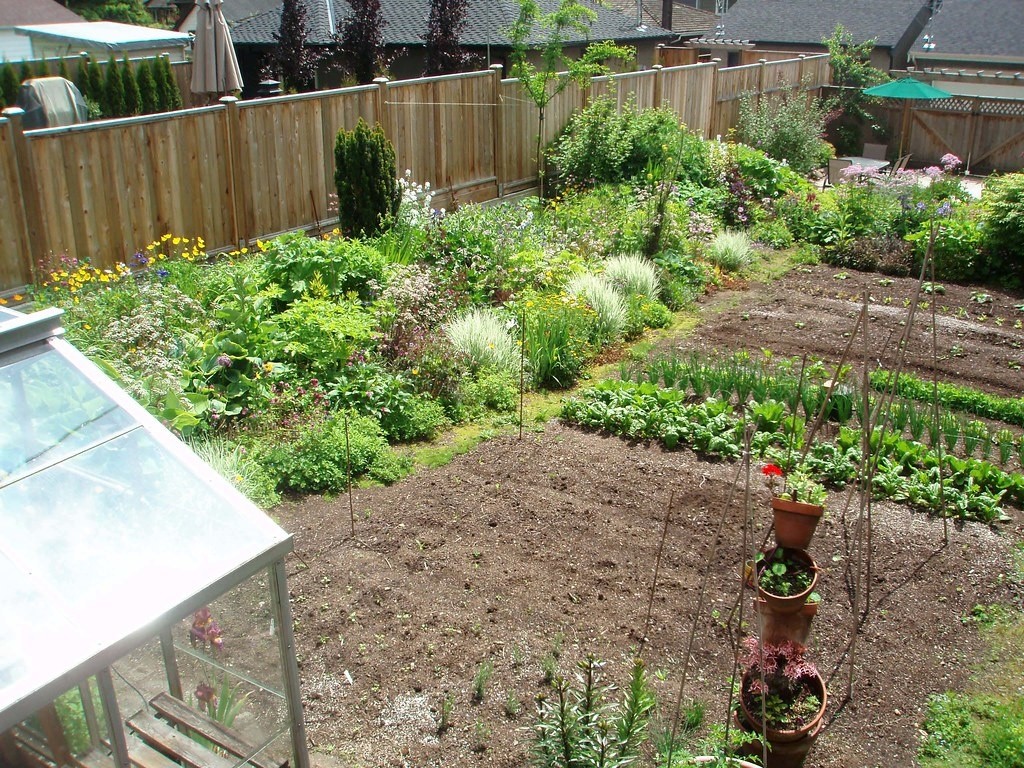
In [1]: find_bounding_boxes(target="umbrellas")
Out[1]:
[191,0,243,100]
[863,76,954,158]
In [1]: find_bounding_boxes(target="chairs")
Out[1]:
[823,158,852,195]
[859,143,888,184]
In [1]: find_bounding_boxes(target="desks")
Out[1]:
[837,157,890,185]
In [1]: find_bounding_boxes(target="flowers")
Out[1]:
[741,638,824,726]
[765,462,826,506]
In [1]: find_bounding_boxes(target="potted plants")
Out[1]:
[729,678,823,765]
[661,723,771,768]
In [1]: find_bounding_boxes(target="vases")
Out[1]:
[743,663,824,743]
[771,500,822,548]
[753,548,817,609]
